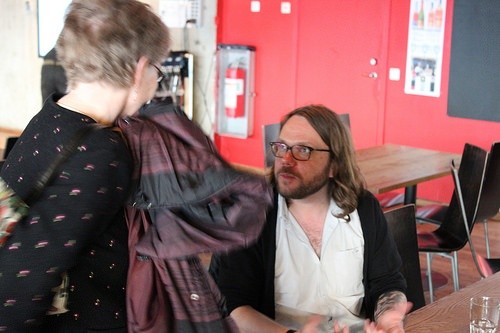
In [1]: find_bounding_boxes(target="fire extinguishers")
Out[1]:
[224,56,248,118]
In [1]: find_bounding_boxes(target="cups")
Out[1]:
[469,296,500,333]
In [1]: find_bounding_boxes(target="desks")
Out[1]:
[350,142,464,211]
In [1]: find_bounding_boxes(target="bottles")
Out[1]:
[436,0,443,28]
[417,0,424,29]
[427,2,435,28]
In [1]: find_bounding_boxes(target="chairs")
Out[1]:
[419,144,493,293]
[413,140,500,258]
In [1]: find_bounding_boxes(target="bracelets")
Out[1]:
[286,329,297,333]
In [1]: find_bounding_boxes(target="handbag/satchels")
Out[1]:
[0,124,132,319]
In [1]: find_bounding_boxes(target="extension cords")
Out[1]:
[186,0,202,29]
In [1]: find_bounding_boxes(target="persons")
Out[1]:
[208,105,407,333]
[0,0,172,333]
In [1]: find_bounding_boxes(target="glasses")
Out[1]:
[148,62,167,84]
[269,141,332,163]
